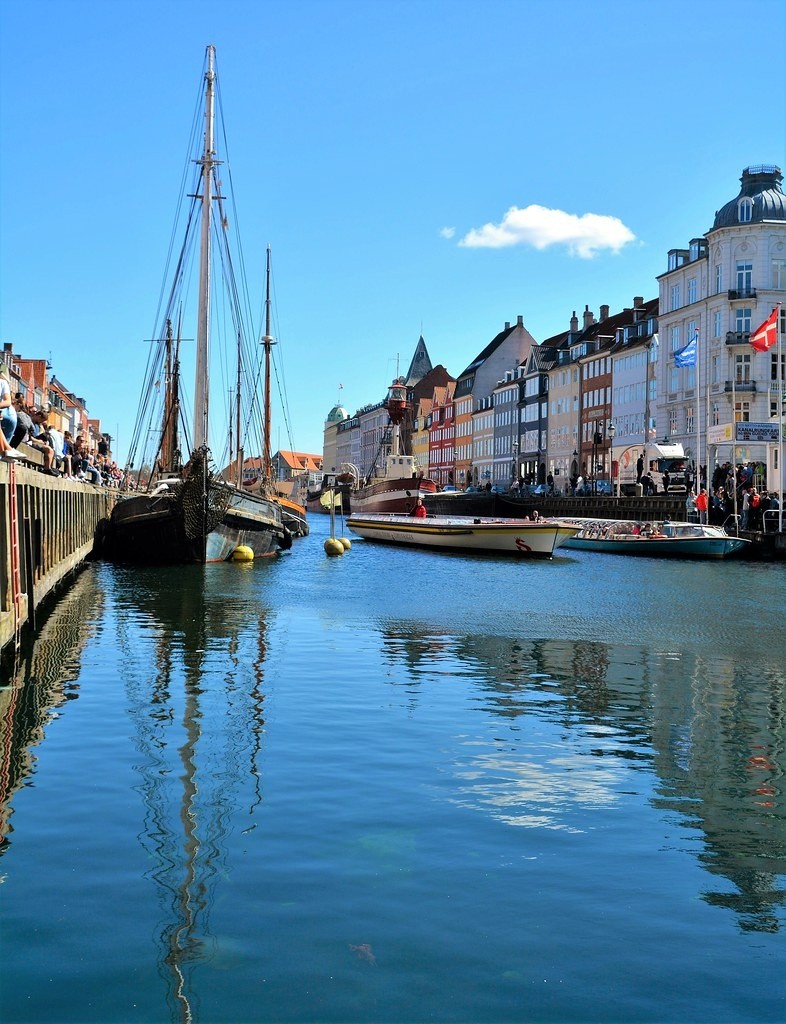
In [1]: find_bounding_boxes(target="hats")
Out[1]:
[666,514,671,517]
[645,524,651,527]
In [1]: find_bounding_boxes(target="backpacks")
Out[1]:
[641,476,647,484]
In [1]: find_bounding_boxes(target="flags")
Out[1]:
[338,383,343,390]
[674,334,698,368]
[748,306,780,352]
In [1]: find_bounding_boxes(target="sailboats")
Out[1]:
[219,242,310,538]
[109,42,294,564]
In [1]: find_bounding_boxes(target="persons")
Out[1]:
[636,454,644,484]
[412,499,427,518]
[449,461,786,542]
[0,363,150,495]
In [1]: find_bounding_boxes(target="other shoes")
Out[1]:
[5,447,27,457]
[0,457,18,463]
[65,472,87,482]
[41,469,60,477]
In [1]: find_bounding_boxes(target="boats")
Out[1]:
[345,352,584,560]
[305,471,358,516]
[538,515,752,562]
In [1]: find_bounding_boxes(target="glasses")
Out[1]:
[80,439,88,444]
[33,410,37,412]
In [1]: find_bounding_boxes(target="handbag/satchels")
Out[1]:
[408,504,418,516]
